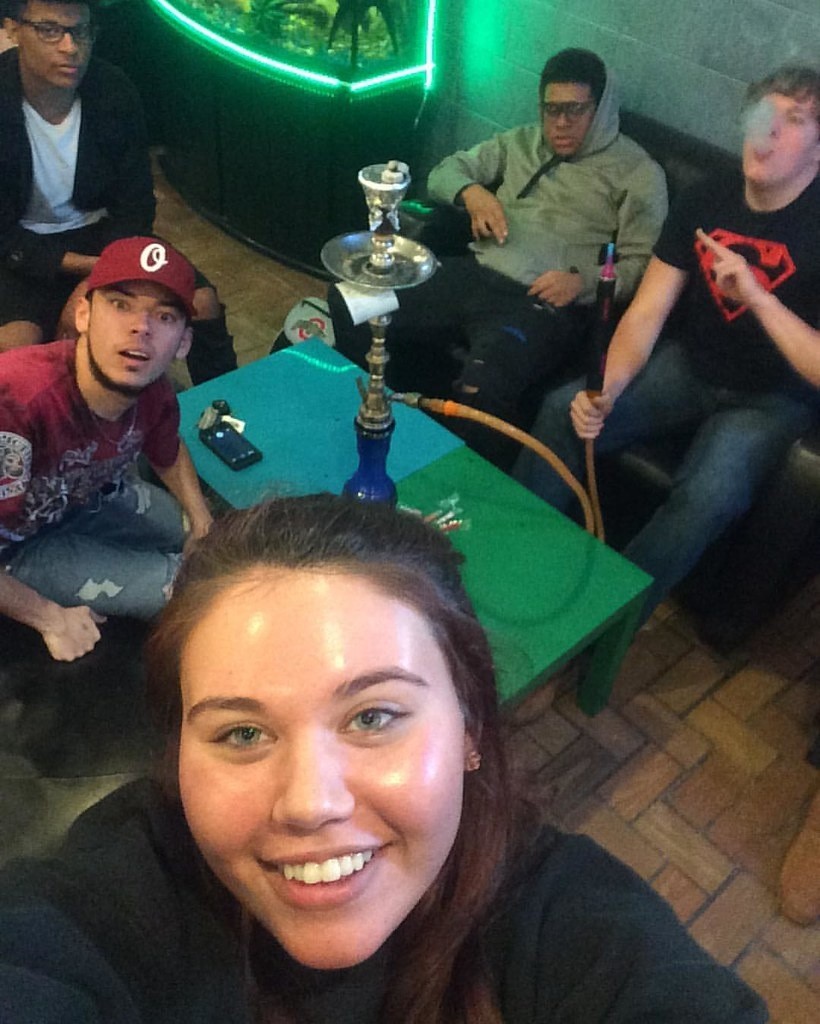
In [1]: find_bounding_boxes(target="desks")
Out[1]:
[176,338,656,723]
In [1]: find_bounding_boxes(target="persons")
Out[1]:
[332,43,671,473]
[1,0,237,386]
[0,238,214,664]
[0,493,774,1024]
[508,58,819,632]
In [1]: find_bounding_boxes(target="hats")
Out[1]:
[86,237,197,319]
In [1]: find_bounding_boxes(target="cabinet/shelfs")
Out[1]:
[147,12,426,282]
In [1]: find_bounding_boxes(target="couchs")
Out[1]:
[329,108,819,653]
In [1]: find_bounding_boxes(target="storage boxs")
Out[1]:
[144,0,431,102]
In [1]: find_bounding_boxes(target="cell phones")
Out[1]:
[200,421,262,471]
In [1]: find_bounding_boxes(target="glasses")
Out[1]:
[15,19,98,43]
[538,98,596,117]
